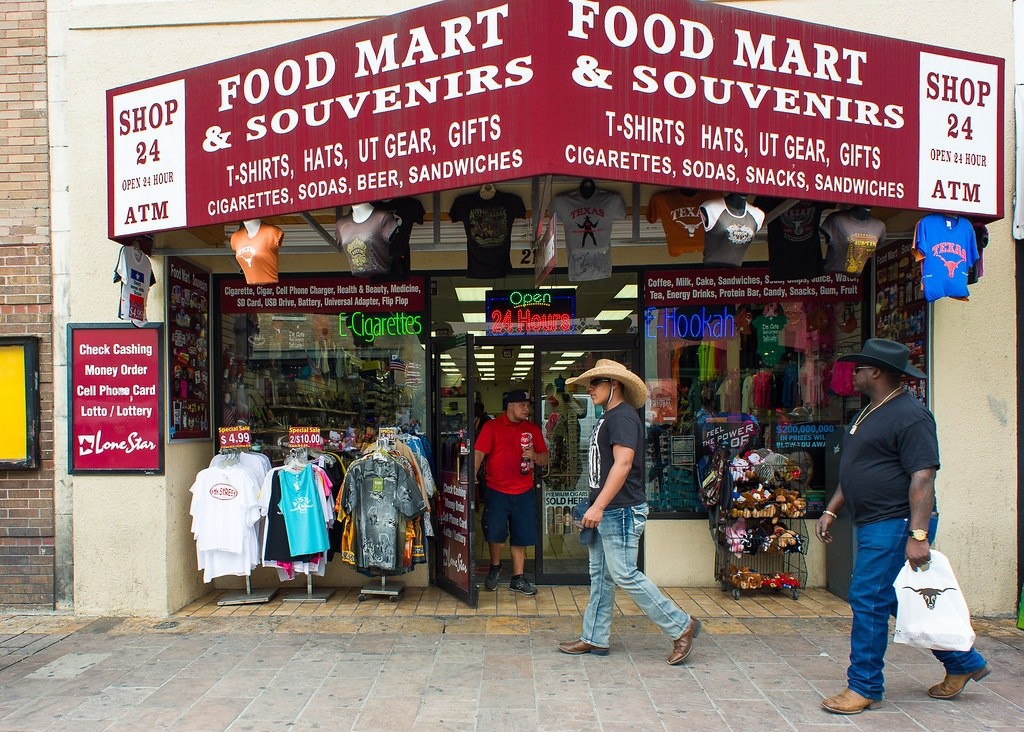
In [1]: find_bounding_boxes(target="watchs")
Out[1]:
[908,528,928,542]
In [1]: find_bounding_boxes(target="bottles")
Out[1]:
[520,449,530,475]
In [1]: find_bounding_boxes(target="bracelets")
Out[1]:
[823,510,838,519]
[533,454,538,462]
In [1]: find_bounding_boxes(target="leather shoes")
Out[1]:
[927,660,992,698]
[558,639,609,656]
[667,616,702,665]
[820,687,883,714]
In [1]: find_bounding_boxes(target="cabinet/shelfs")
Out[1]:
[710,445,812,594]
[244,387,416,437]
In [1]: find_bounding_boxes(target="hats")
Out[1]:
[837,338,928,378]
[507,390,533,403]
[565,358,647,408]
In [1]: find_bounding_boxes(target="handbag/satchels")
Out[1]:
[893,550,975,652]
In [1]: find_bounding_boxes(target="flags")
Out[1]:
[390,355,406,372]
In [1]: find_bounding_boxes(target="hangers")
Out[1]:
[132,238,143,253]
[283,447,325,469]
[363,436,393,461]
[579,179,596,194]
[213,447,249,470]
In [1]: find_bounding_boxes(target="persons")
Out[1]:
[234,219,282,284]
[334,203,398,276]
[696,192,764,266]
[557,359,701,665]
[474,390,549,596]
[821,203,886,274]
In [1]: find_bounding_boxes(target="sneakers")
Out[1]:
[508,577,538,595]
[485,565,502,590]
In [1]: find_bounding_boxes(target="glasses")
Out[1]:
[854,365,883,374]
[590,377,613,386]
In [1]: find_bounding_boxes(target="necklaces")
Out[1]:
[849,386,901,435]
